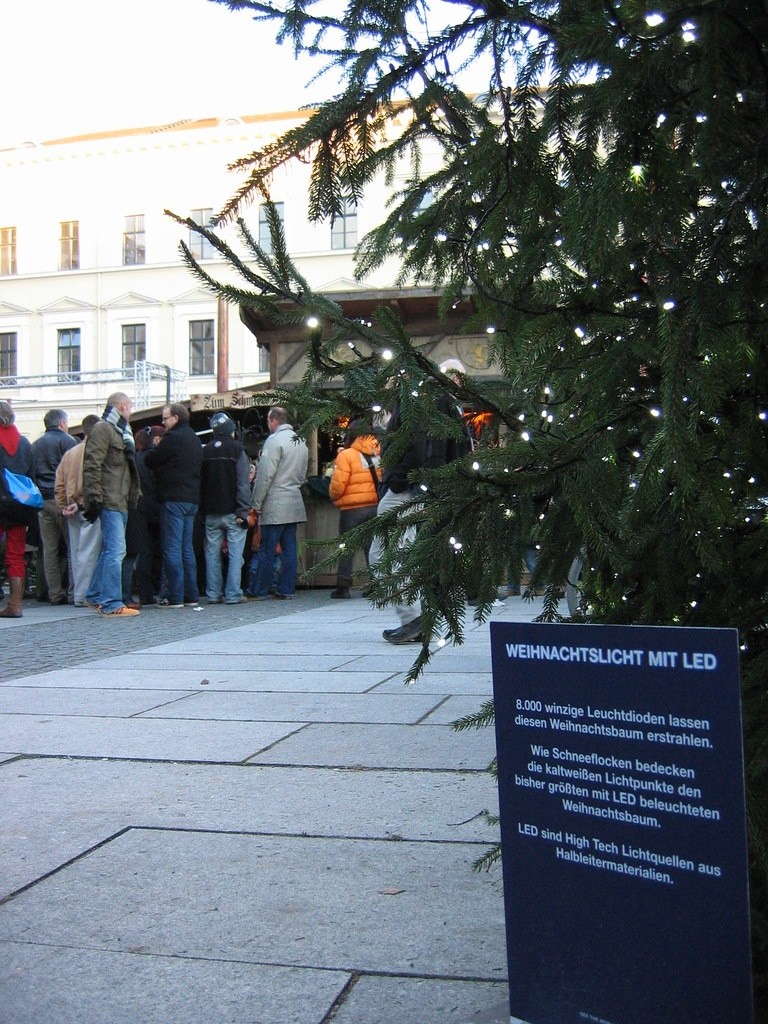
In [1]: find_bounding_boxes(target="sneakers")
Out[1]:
[101,605,140,618]
[95,604,103,612]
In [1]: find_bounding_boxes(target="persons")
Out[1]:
[244,406,310,601]
[144,401,202,608]
[122,424,283,610]
[82,392,141,616]
[53,414,100,607]
[0,402,37,618]
[200,412,251,603]
[328,421,385,599]
[365,364,546,643]
[31,409,78,606]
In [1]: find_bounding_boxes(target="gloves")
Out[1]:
[82,501,101,524]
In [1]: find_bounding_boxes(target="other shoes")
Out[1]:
[52,597,67,605]
[225,596,248,603]
[382,616,425,643]
[184,599,199,606]
[331,587,350,598]
[467,592,509,606]
[362,584,380,597]
[530,588,543,596]
[272,591,294,600]
[158,598,180,608]
[140,598,157,605]
[556,588,565,597]
[502,590,520,596]
[24,591,36,598]
[38,596,48,602]
[243,592,267,601]
[74,602,89,606]
[209,599,222,604]
[123,598,142,610]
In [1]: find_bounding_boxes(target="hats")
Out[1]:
[210,412,236,436]
[350,420,372,434]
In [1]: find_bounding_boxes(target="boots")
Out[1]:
[0,577,24,617]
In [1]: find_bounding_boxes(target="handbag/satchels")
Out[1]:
[0,468,45,526]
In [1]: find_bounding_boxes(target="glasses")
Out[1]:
[161,415,172,421]
